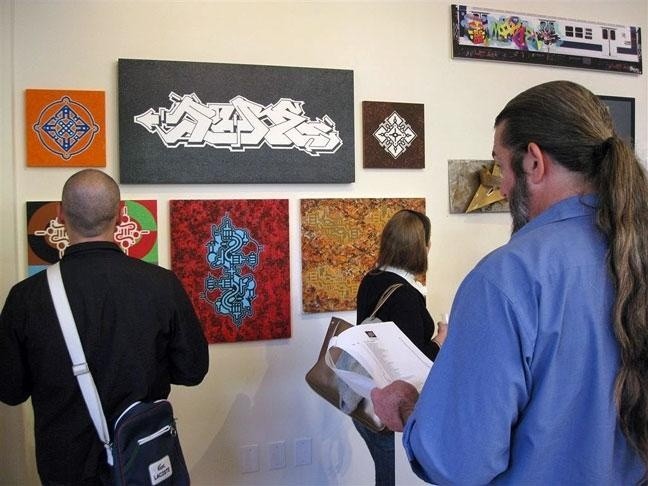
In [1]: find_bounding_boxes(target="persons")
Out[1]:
[371,81,648,485]
[0,170,209,486]
[351,210,449,486]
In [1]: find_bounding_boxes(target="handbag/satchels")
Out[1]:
[109,399,191,486]
[305,281,418,435]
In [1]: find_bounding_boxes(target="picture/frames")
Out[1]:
[595,95,635,154]
[448,6,645,75]
